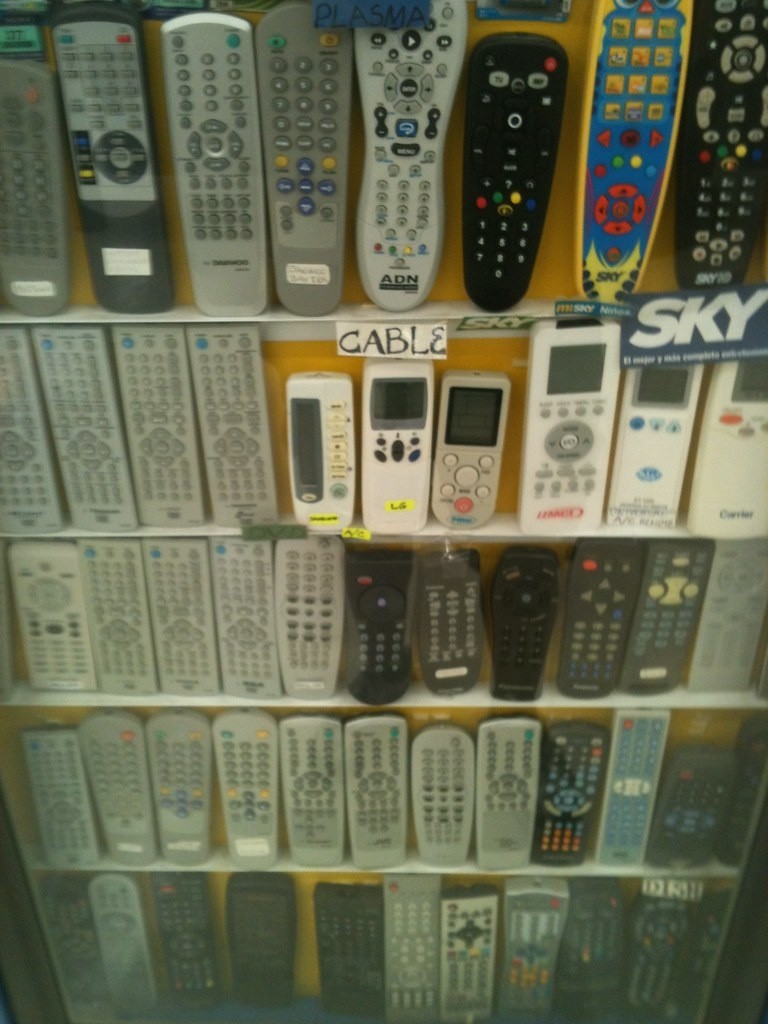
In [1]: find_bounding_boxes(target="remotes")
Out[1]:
[0,0,768,1024]
